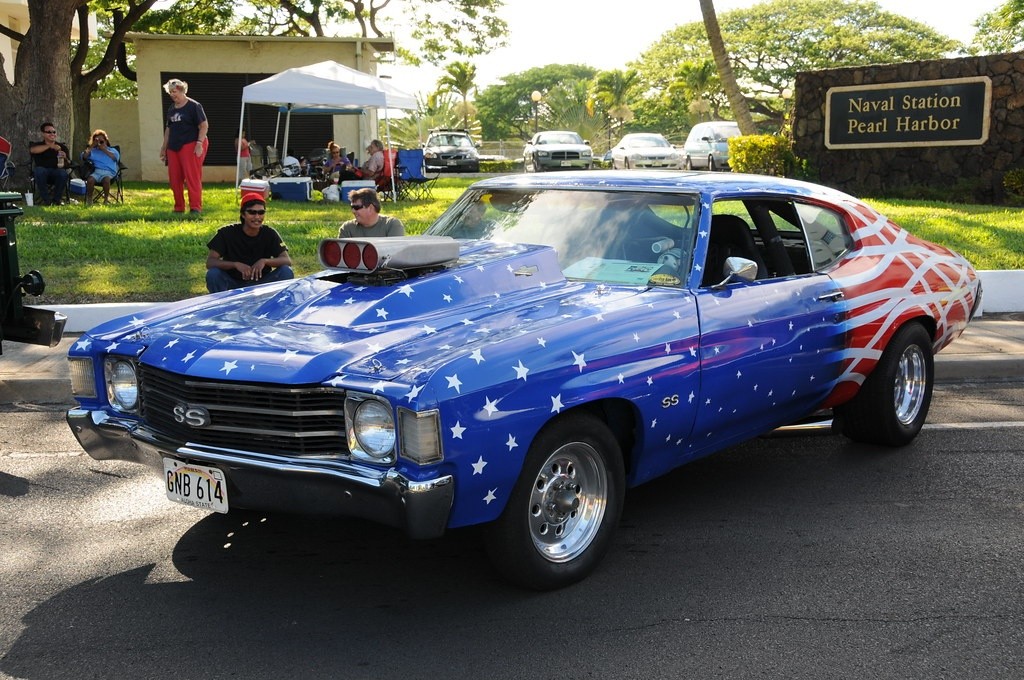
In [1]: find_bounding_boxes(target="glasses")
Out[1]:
[350,204,365,210]
[243,209,265,215]
[44,130,56,134]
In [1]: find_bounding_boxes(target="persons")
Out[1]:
[235,127,252,183]
[160,79,208,221]
[28,123,71,207]
[324,142,351,179]
[283,147,310,177]
[80,130,120,206]
[360,140,385,180]
[338,188,404,238]
[206,193,294,294]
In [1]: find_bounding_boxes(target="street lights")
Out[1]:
[531,90,541,132]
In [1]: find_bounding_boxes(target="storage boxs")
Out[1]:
[342,178,375,201]
[269,177,315,200]
[242,176,267,199]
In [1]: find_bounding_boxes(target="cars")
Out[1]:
[611,132,681,169]
[523,128,594,171]
[66,172,982,593]
[684,121,743,171]
[422,129,481,172]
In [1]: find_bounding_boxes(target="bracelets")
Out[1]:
[196,140,202,144]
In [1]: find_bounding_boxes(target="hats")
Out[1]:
[241,193,266,208]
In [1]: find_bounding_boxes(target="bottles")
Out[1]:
[57,151,65,167]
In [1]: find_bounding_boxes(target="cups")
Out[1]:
[25,193,33,206]
[334,177,339,184]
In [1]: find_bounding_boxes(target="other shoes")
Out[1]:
[52,197,64,206]
[189,209,201,219]
[104,201,110,205]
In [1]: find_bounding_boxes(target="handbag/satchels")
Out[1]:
[323,185,341,203]
[337,164,366,184]
[78,159,95,180]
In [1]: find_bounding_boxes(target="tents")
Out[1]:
[235,60,423,203]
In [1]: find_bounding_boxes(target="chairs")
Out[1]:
[0,136,16,188]
[28,139,78,203]
[695,214,769,286]
[82,145,126,204]
[322,148,441,204]
